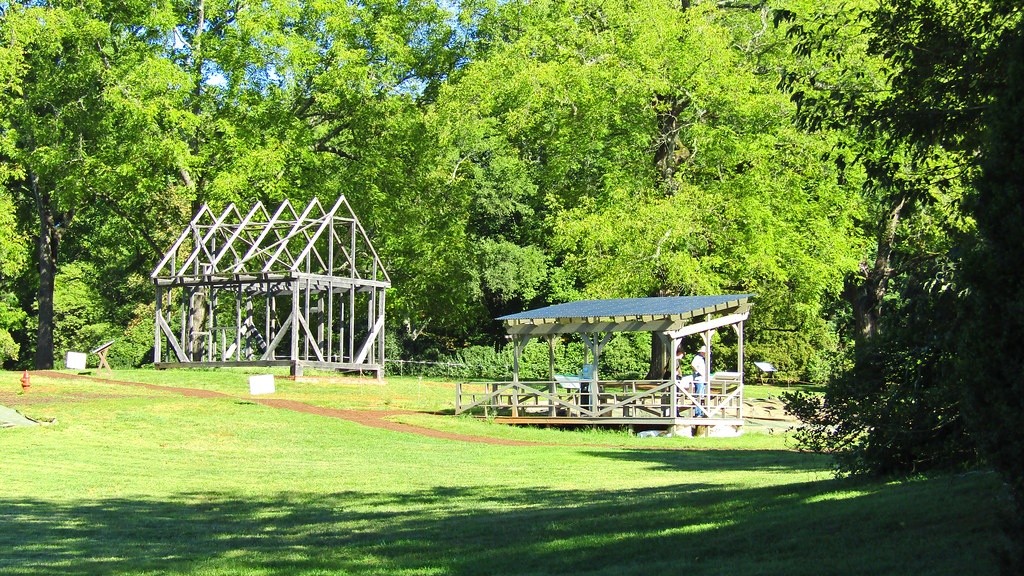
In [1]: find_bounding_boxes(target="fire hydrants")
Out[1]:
[20,369,31,391]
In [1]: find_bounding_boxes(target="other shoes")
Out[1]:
[677,412,685,417]
[693,414,704,418]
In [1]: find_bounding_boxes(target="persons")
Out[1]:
[690,345,709,419]
[663,346,686,417]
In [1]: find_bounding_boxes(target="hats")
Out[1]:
[676,344,686,353]
[697,345,706,352]
